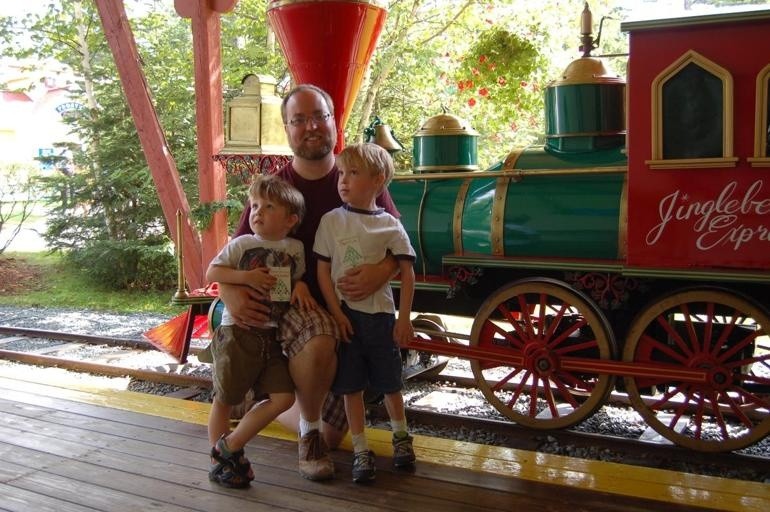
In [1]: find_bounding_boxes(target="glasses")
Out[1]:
[284,113,332,128]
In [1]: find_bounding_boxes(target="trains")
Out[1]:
[140,0,768,454]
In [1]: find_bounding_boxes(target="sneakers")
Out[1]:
[298,429,336,480]
[392,431,416,466]
[352,450,376,483]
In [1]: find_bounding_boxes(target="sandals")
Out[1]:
[209,433,256,488]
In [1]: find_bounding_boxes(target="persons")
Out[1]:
[205,174,307,489]
[312,142,417,484]
[231,84,402,482]
